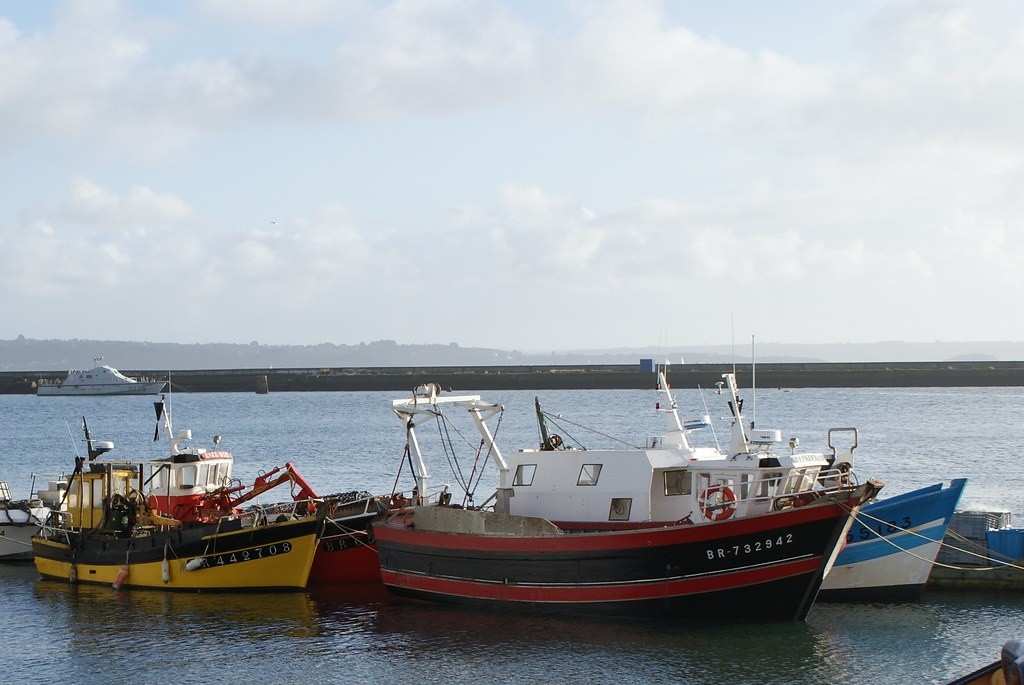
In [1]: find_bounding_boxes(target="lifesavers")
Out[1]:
[697,483,738,521]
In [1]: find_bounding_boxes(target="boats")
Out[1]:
[783,390,790,392]
[142,428,412,587]
[0,475,68,562]
[815,478,970,604]
[37,365,169,396]
[924,528,1023,592]
[31,417,342,594]
[370,372,884,625]
